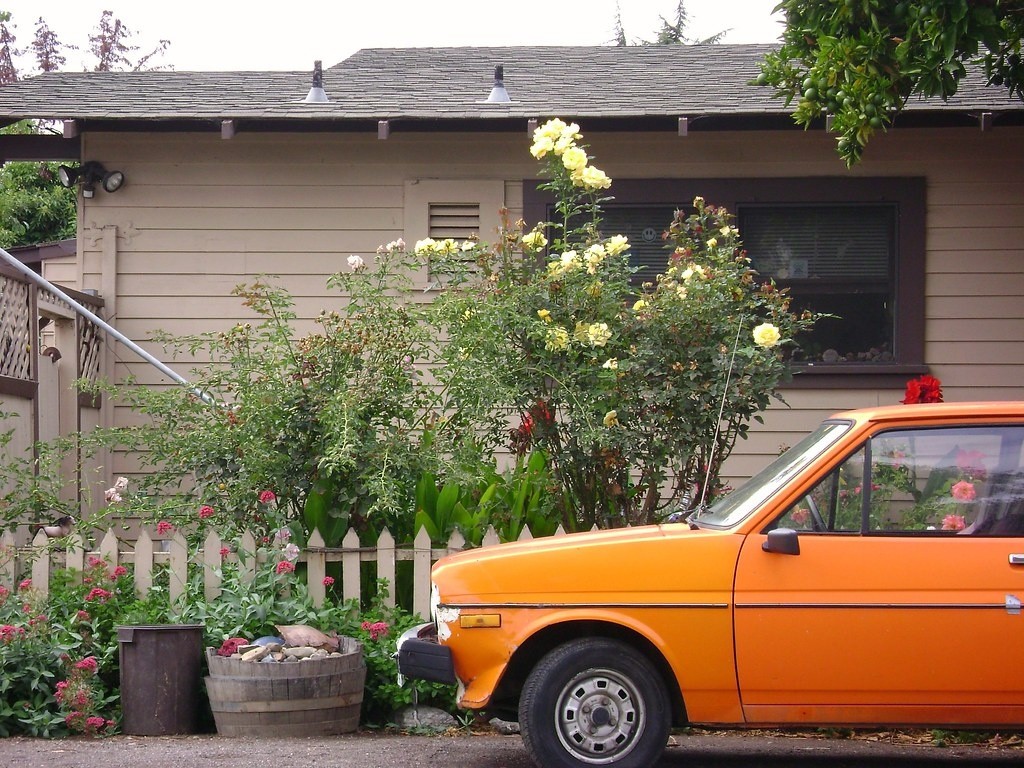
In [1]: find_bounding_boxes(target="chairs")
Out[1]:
[954,467,1024,538]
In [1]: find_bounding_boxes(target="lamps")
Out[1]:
[57,161,125,199]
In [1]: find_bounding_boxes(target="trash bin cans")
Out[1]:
[114,621,206,734]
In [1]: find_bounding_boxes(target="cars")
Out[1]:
[390,401,1024,768]
